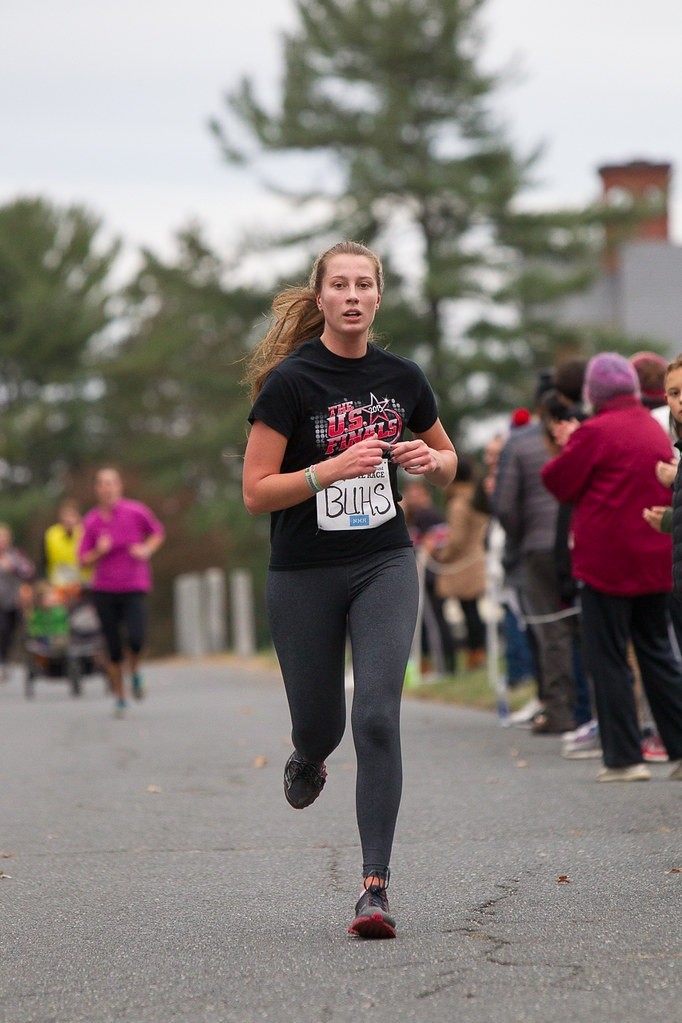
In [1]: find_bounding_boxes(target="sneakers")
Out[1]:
[284,749,327,809]
[348,866,397,937]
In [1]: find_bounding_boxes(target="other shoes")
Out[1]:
[508,695,541,723]
[563,737,603,759]
[530,703,590,733]
[670,761,682,780]
[643,738,669,761]
[596,764,650,782]
[115,698,128,719]
[562,722,600,741]
[131,675,143,698]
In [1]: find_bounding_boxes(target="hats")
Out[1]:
[586,351,640,400]
[629,352,667,393]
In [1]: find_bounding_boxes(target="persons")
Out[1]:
[242,241,457,936]
[400,351,682,783]
[0,467,165,715]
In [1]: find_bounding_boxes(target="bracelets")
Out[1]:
[305,465,323,493]
